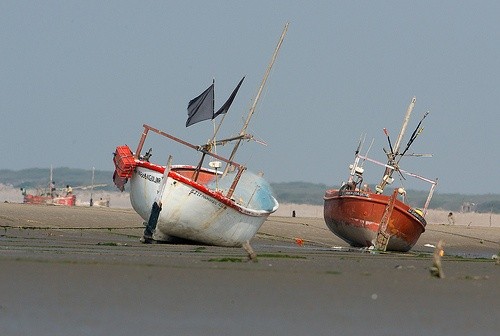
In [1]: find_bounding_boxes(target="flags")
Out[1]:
[185,84,215,128]
[211,76,245,120]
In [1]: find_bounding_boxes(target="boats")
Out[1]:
[128,22,289,245]
[23,165,76,207]
[76,167,110,207]
[323,98,438,255]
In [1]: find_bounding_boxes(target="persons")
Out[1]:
[65,185,72,197]
[21,187,26,195]
[50,181,57,194]
